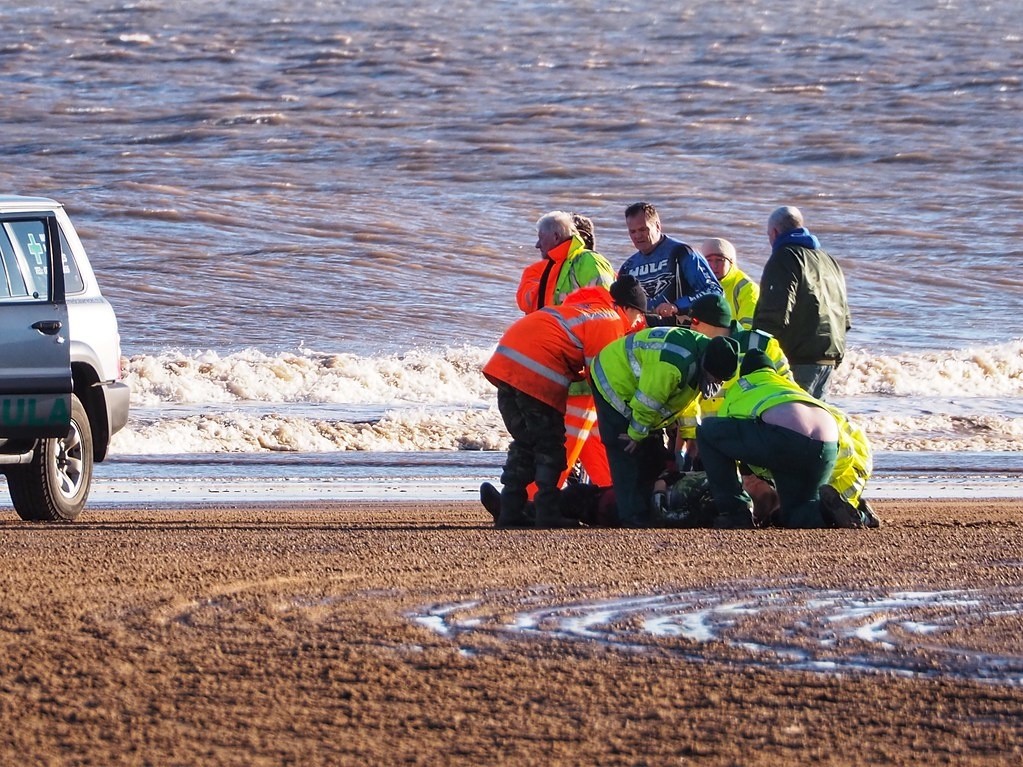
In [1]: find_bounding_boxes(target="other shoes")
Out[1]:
[622,510,665,528]
[497,509,534,529]
[819,483,853,528]
[713,512,733,528]
[858,499,881,528]
[534,511,579,529]
[480,482,502,520]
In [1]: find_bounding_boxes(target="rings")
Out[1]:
[663,310,667,312]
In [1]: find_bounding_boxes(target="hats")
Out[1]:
[704,336,740,381]
[574,215,594,240]
[610,274,648,313]
[688,293,732,328]
[702,238,736,263]
[739,348,777,378]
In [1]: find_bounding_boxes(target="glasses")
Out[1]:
[584,239,592,245]
[714,376,722,383]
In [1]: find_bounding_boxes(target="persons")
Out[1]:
[753,205,851,403]
[479,273,647,530]
[616,201,724,329]
[695,347,864,530]
[589,325,741,528]
[479,202,881,530]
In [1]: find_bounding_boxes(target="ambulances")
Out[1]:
[0,195,129,522]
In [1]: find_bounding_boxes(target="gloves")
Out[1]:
[656,471,686,487]
